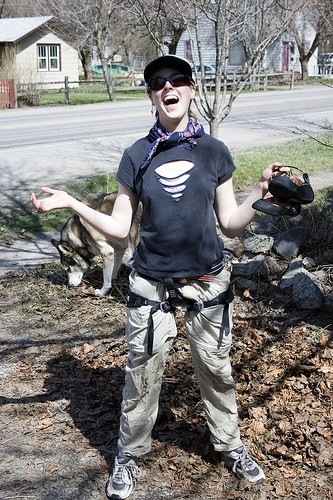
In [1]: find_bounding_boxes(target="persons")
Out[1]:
[30,54,305,500]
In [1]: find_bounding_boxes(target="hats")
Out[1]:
[141,54,197,83]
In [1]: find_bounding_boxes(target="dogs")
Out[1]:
[50,191,143,296]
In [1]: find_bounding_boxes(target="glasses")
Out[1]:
[148,73,194,92]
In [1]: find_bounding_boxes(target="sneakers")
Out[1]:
[223,445,266,485]
[105,454,142,499]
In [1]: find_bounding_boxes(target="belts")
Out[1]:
[137,262,224,284]
